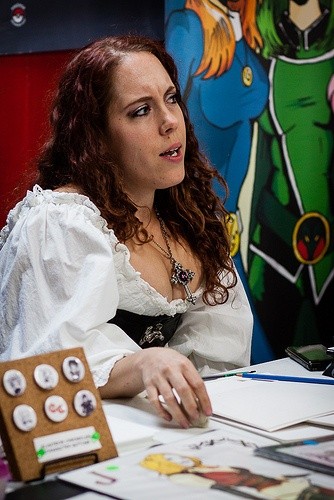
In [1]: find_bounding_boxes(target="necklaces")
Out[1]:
[143,212,197,307]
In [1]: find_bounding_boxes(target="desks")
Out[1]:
[0,356,333,500]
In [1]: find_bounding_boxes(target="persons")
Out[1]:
[0,37,255,429]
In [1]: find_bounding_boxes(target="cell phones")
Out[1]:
[285,344,333,371]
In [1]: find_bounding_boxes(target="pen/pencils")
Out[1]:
[202,370,256,382]
[236,374,334,385]
